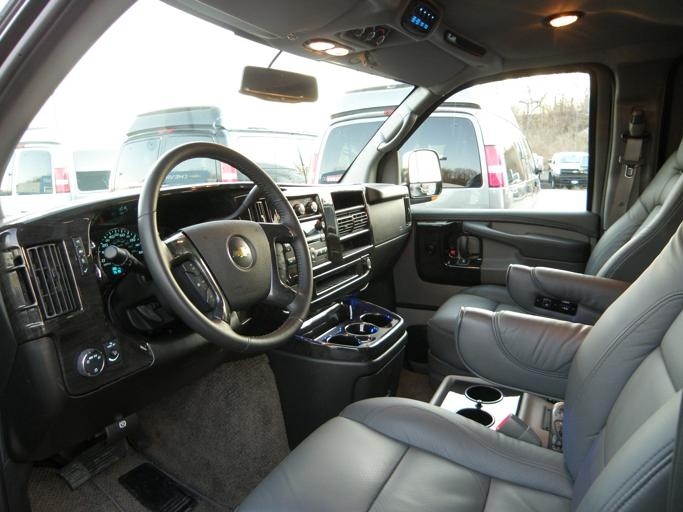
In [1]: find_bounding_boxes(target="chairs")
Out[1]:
[424,142,682,397]
[232,219,681,510]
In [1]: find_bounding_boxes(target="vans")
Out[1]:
[316,82,543,212]
[110,104,321,191]
[0,135,115,222]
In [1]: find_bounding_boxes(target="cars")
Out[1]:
[547,151,588,188]
